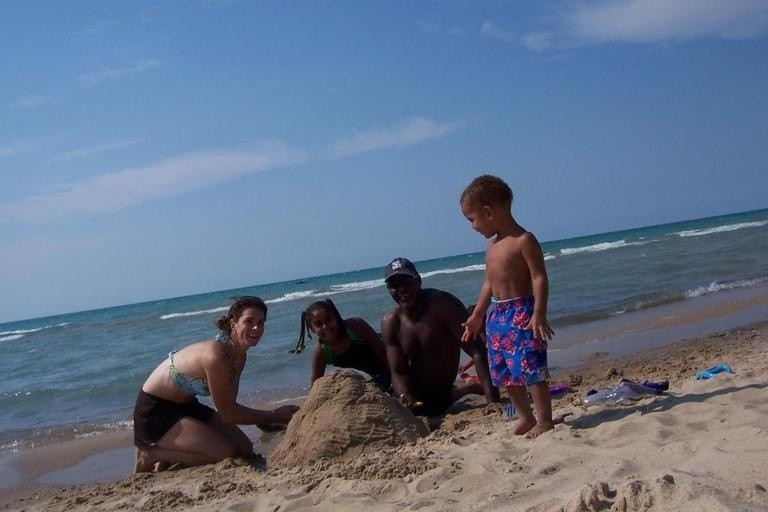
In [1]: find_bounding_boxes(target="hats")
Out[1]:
[385,257,418,280]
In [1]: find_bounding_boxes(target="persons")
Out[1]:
[381,257,503,418]
[459,173,556,438]
[458,304,486,383]
[288,299,400,400]
[133,295,297,473]
